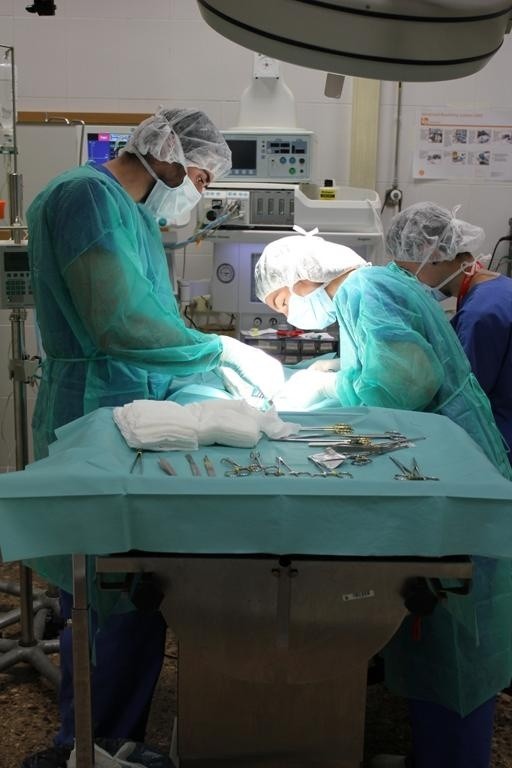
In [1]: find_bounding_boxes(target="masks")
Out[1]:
[143,150,202,228]
[415,248,461,301]
[286,282,337,330]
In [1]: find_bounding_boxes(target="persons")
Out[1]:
[24,107,288,767]
[253,223,512,768]
[381,198,512,466]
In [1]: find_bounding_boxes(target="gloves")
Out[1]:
[218,335,285,408]
[277,357,341,411]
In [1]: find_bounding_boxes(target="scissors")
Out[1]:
[270,422,426,466]
[220,452,355,478]
[389,455,441,481]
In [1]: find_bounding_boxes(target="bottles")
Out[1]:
[320,179,335,200]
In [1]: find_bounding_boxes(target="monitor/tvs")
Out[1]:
[74,123,136,174]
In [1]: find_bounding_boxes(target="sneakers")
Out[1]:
[56,736,170,767]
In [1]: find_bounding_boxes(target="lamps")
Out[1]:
[194,0,511,83]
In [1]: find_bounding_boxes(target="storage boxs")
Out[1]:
[293,184,381,232]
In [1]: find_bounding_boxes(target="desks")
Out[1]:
[1,406,512,768]
